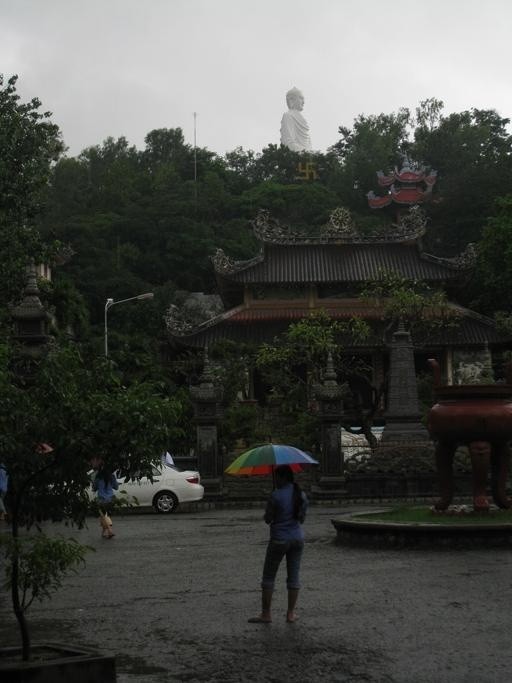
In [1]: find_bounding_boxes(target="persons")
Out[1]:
[92,460,118,539]
[248,464,308,623]
[0,461,10,520]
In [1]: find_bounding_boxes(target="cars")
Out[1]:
[48,458,205,516]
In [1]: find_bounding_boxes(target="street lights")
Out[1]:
[103,292,156,359]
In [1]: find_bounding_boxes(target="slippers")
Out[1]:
[286,613,301,623]
[247,615,272,623]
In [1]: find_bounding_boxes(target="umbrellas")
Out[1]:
[226,434,320,491]
